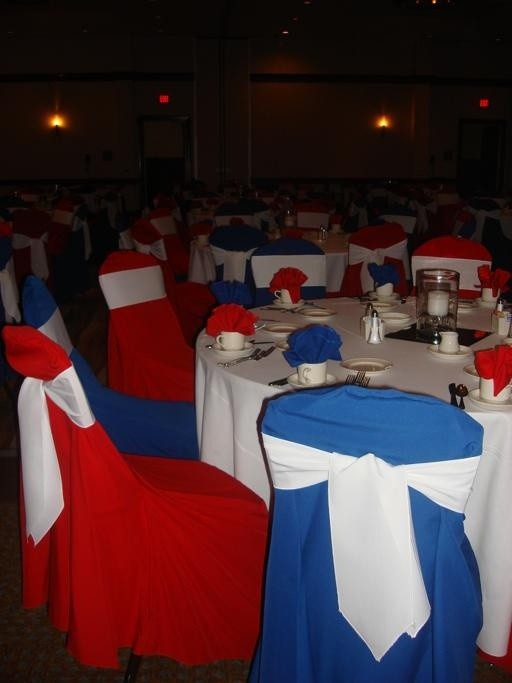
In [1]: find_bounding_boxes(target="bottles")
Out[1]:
[366,302,381,345]
[496,297,504,312]
[284,196,297,226]
[317,223,328,242]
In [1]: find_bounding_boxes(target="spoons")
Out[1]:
[456,382,468,413]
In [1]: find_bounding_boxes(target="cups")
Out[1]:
[274,289,293,304]
[438,331,460,353]
[376,282,394,297]
[296,361,328,386]
[481,287,501,302]
[217,331,245,351]
[480,377,512,403]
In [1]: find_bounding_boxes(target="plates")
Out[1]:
[475,298,497,307]
[362,301,399,312]
[379,313,411,324]
[212,342,255,353]
[463,364,480,379]
[468,388,512,410]
[262,324,299,336]
[338,357,393,376]
[368,292,400,299]
[427,344,473,358]
[286,375,339,390]
[298,307,338,319]
[273,298,305,308]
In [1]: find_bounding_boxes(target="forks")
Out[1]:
[344,368,371,388]
